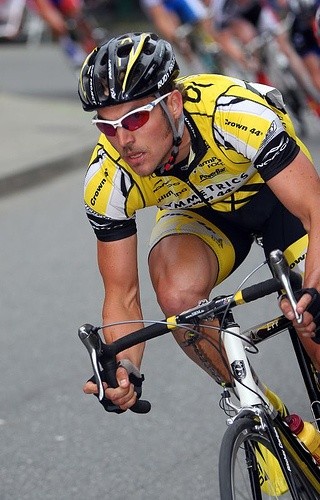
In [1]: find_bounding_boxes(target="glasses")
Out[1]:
[92,93,171,137]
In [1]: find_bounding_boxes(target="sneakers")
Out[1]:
[254,389,291,497]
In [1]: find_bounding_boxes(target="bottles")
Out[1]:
[288,414,320,464]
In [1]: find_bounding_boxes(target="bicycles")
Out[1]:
[77,247,319,500]
[57,5,311,139]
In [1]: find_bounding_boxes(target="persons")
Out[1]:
[1,0,320,139]
[76,31,320,415]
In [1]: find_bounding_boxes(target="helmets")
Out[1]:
[77,33,181,111]
